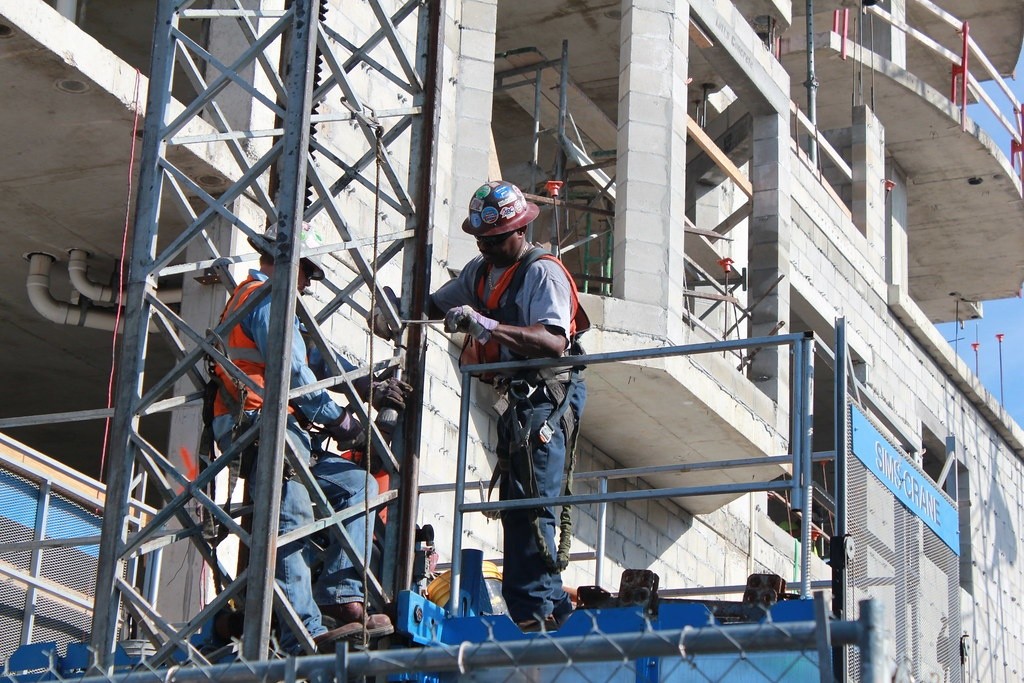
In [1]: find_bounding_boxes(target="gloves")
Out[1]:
[325,406,369,452]
[358,372,412,409]
[443,305,499,344]
[367,286,399,337]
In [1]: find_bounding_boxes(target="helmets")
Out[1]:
[248,218,325,280]
[462,181,541,236]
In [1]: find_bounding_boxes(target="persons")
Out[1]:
[209,223,412,655]
[367,184,584,634]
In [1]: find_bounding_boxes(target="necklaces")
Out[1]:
[486,242,529,291]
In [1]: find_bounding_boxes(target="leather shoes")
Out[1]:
[299,623,371,654]
[317,599,394,636]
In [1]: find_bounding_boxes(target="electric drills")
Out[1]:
[338,369,403,473]
